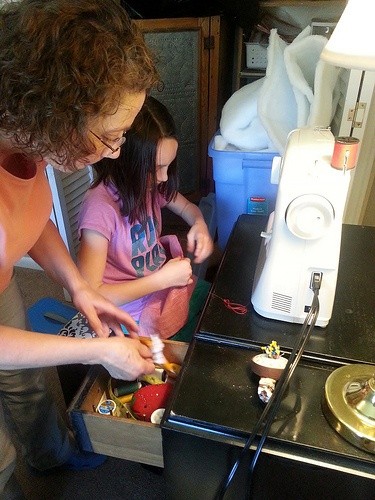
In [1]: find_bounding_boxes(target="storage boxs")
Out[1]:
[243,41,269,70]
[208,127,280,250]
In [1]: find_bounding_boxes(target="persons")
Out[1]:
[0,0,155,500]
[77,96,214,343]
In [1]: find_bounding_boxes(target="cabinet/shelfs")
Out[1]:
[64,213,375,500]
[128,15,220,204]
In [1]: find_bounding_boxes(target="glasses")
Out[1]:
[86,127,126,158]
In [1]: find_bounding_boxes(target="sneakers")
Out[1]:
[29,449,107,472]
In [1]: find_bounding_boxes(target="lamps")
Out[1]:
[319,0,375,72]
